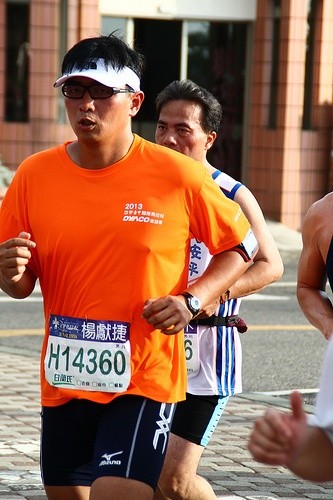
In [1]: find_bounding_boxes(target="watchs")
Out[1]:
[176,292,201,318]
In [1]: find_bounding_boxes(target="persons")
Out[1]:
[297,193,333,337]
[155,79,284,500]
[248,336,333,483]
[1,28,259,500]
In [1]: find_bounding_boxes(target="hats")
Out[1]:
[53,57,140,93]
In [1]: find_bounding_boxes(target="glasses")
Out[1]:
[62,82,135,99]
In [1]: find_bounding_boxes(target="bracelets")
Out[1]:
[225,289,230,300]
[221,295,225,304]
